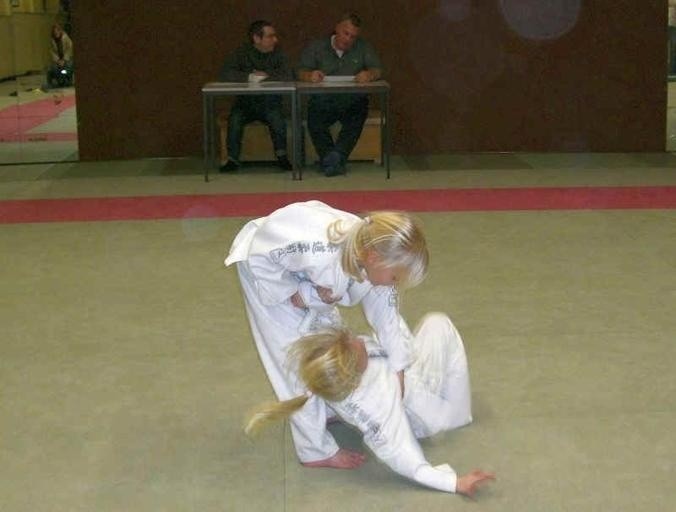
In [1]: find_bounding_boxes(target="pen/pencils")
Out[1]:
[318,73,324,81]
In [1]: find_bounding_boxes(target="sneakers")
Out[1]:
[322,152,346,177]
[277,153,293,171]
[218,159,239,173]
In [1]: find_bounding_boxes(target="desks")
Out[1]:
[199,75,392,185]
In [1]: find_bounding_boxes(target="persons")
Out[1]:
[44,23,74,89]
[288,281,495,500]
[217,19,295,171]
[224,199,432,469]
[294,12,392,177]
